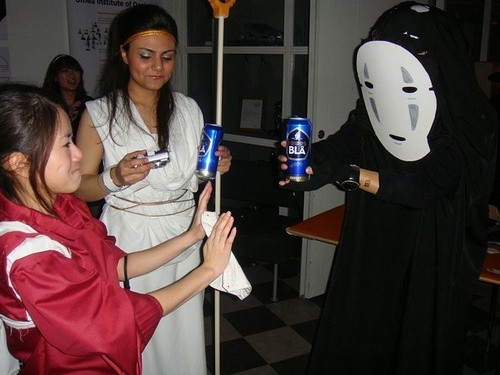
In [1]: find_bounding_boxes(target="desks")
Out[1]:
[286,204,500,367]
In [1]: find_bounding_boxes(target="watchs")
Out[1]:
[340,163,360,192]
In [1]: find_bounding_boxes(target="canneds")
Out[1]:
[196,123,224,180]
[286,117,312,182]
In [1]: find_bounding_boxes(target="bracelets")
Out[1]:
[97,164,132,193]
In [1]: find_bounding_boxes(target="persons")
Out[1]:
[273,1,490,374]
[0,81,236,375]
[43,54,95,147]
[70,4,232,374]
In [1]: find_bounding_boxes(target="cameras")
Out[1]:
[134,151,170,169]
